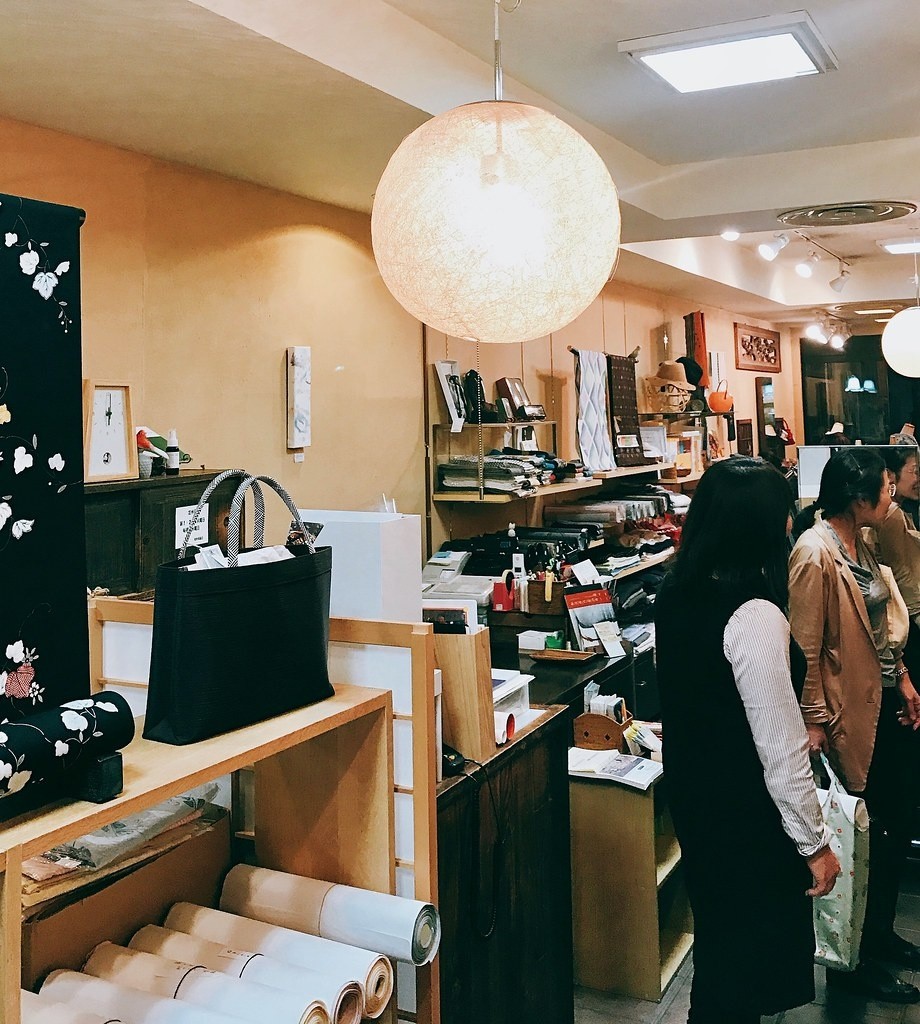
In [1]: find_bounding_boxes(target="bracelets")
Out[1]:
[895,667,909,677]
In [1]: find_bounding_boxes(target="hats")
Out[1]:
[649,361,697,391]
[674,357,703,386]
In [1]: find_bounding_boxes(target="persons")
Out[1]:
[818,422,853,445]
[652,453,844,1023]
[888,423,918,445]
[784,441,919,1004]
[878,447,920,860]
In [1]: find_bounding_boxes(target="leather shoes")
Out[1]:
[822,964,920,1005]
[860,930,920,969]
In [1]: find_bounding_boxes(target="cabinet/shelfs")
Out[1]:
[436,330,851,1024]
[0,680,403,1023]
[83,470,246,594]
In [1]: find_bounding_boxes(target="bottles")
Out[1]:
[165,427,180,476]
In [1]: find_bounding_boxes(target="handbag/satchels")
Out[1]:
[806,746,870,973]
[142,470,336,747]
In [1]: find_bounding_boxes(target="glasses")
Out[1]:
[880,482,897,499]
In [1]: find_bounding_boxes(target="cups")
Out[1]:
[139,453,152,479]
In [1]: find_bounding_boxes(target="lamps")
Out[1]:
[806,319,825,338]
[832,330,852,347]
[368,0,619,343]
[758,235,791,260]
[795,251,822,279]
[830,271,850,290]
[817,324,838,344]
